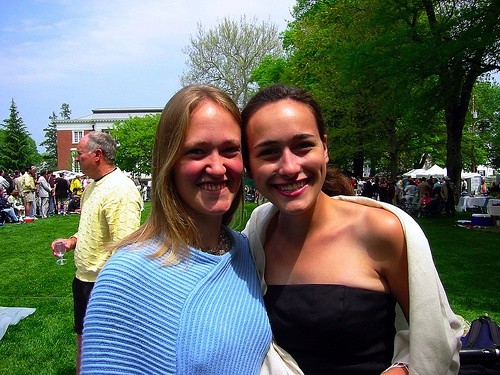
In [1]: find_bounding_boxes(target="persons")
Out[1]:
[0,163,152,226]
[239,87,459,375]
[78,83,273,375]
[346,172,500,222]
[51,130,144,375]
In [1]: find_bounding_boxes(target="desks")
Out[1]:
[455,196,500,216]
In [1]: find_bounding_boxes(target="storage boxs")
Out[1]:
[24,218,34,222]
[471,214,491,227]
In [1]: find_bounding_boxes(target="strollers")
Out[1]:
[458,316,500,375]
[414,197,439,217]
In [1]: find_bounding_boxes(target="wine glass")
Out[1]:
[53,240,68,266]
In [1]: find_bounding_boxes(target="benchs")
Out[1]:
[405,203,417,212]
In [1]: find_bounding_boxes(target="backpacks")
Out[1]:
[460,315,500,351]
